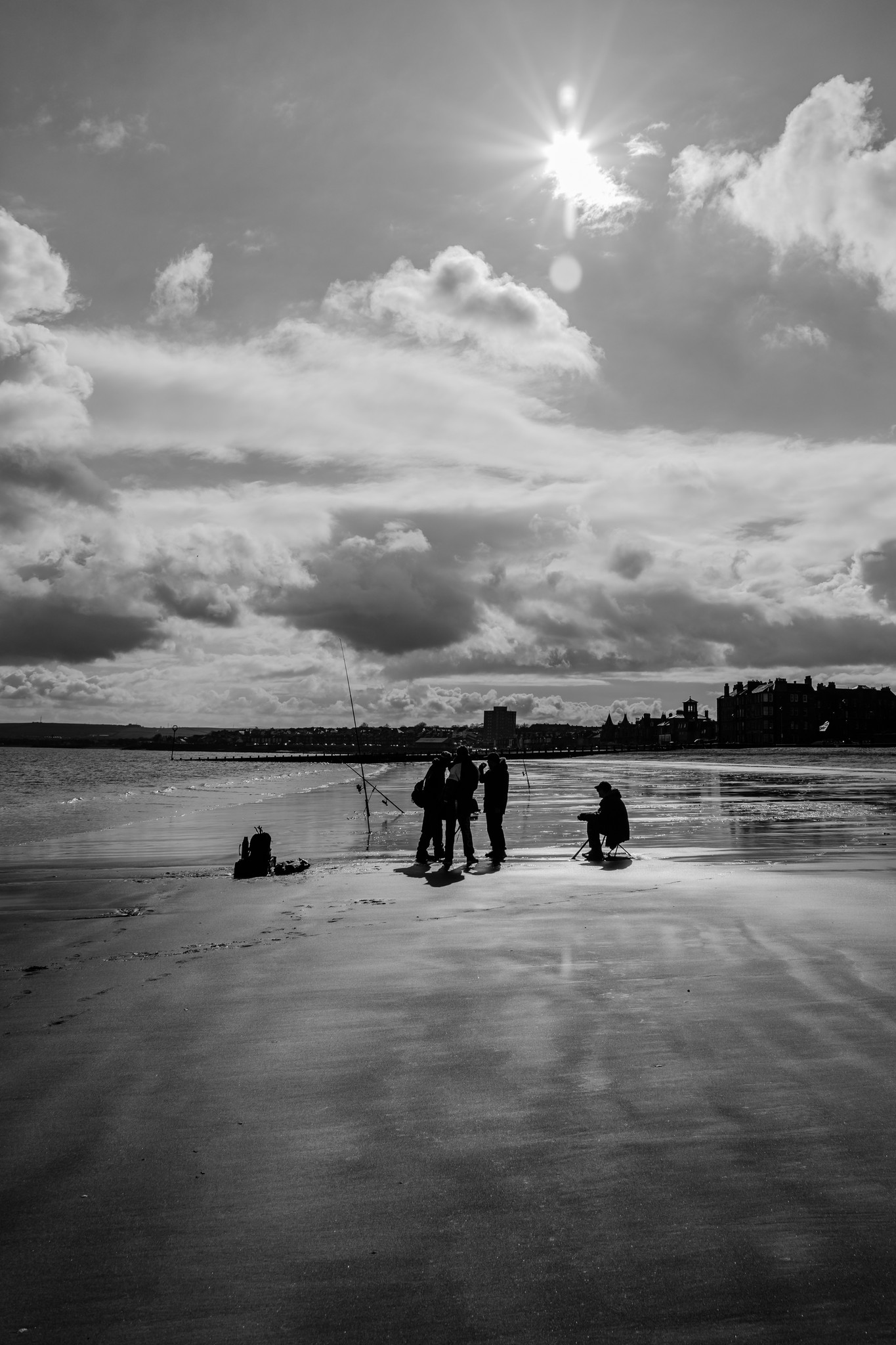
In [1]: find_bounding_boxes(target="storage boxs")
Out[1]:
[233,856,277,878]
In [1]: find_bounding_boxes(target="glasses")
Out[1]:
[596,789,602,793]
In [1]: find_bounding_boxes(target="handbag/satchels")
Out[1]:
[472,799,478,813]
[411,764,445,808]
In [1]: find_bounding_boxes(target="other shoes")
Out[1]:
[485,848,493,857]
[466,854,478,863]
[584,851,604,860]
[434,851,445,858]
[416,853,434,862]
[491,849,507,866]
[442,858,452,864]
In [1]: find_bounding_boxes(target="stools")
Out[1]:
[597,831,634,859]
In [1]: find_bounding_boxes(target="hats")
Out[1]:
[440,751,452,763]
[456,745,469,755]
[594,781,612,792]
[483,752,499,760]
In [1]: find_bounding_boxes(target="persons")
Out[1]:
[442,745,479,863]
[577,781,629,859]
[415,751,451,859]
[479,752,509,860]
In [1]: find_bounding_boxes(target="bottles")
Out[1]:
[241,837,249,860]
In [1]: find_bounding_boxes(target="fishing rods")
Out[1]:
[517,740,530,785]
[338,638,372,832]
[338,758,405,814]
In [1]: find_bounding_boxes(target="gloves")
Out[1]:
[577,813,586,821]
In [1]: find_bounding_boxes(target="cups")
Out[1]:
[482,762,487,768]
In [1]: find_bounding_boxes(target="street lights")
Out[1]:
[170,724,178,760]
